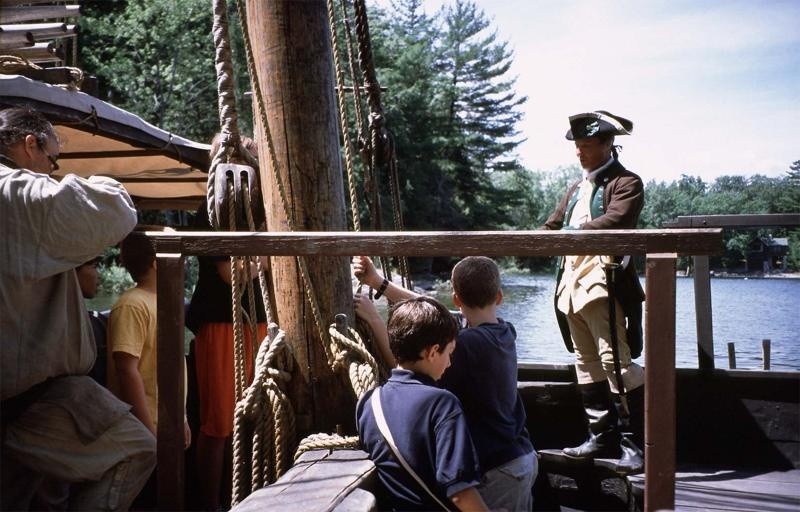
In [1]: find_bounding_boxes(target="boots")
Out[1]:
[611,382,646,476]
[561,378,623,460]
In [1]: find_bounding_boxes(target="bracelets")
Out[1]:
[374,279,389,300]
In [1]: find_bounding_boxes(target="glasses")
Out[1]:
[37,137,59,175]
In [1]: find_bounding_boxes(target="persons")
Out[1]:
[106,225,195,511]
[353,295,489,511]
[0,99,159,512]
[353,254,541,512]
[533,108,650,476]
[75,252,108,391]
[184,130,275,511]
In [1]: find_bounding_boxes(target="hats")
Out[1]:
[565,109,633,141]
